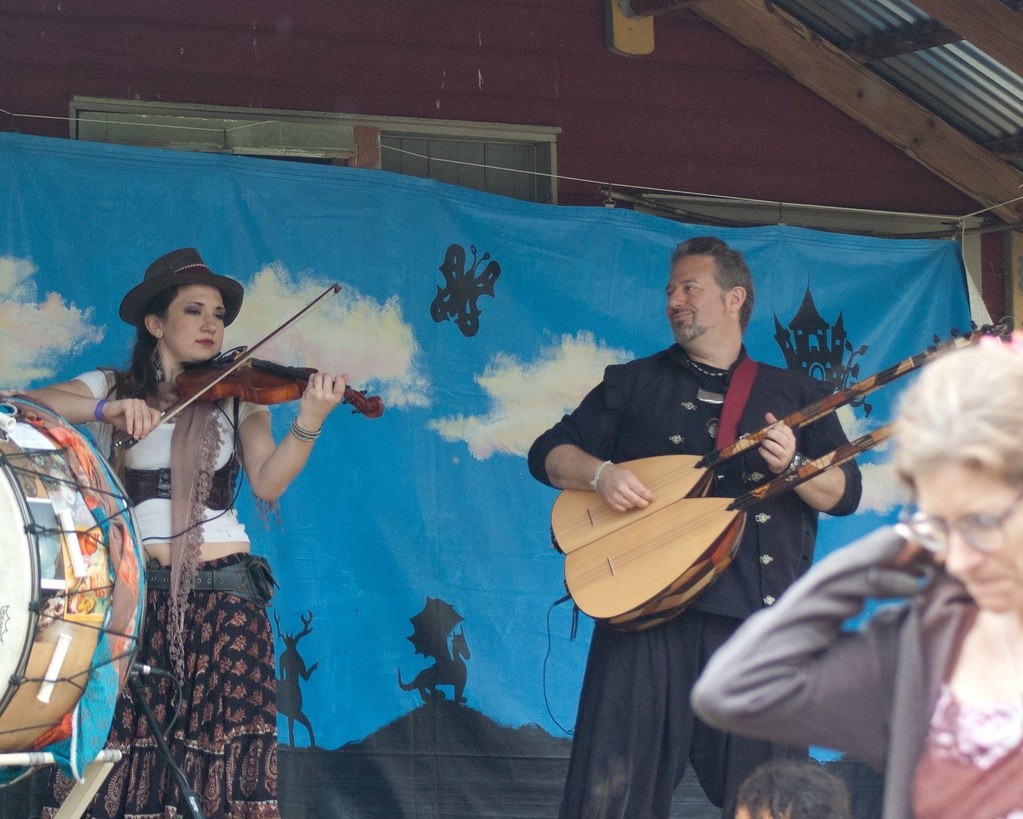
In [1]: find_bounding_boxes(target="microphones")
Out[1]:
[132,661,170,678]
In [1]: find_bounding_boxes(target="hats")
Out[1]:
[120,248,244,327]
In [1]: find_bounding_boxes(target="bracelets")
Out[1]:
[787,452,806,471]
[292,417,320,441]
[94,400,110,421]
[590,460,615,492]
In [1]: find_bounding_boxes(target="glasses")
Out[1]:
[895,493,1023,557]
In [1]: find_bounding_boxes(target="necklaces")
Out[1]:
[691,361,729,376]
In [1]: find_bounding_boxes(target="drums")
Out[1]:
[2,387,148,789]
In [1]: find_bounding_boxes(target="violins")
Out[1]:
[174,351,386,418]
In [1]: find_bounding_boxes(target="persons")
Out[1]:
[27,249,348,819]
[735,758,851,819]
[528,235,861,819]
[690,326,1023,819]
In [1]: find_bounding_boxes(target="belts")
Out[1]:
[146,557,262,608]
